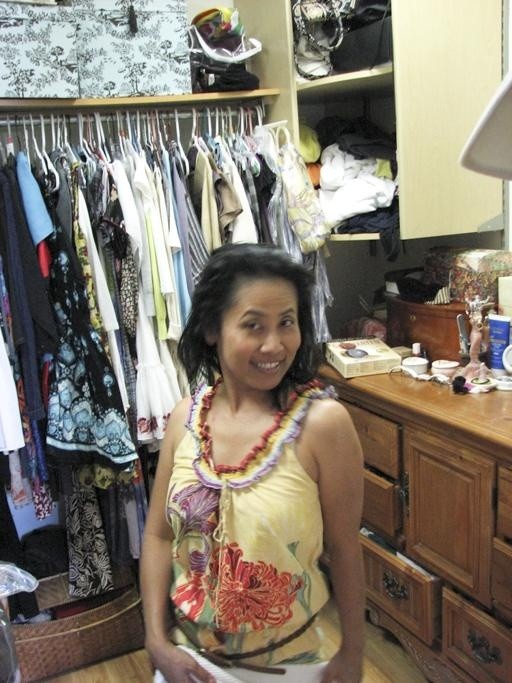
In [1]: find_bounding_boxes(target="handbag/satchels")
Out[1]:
[290,0,391,85]
[188,5,262,92]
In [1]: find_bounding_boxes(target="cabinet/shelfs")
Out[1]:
[233,0,505,242]
[309,339,512,682]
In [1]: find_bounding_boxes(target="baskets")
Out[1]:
[1,564,145,683]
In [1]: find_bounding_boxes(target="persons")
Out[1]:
[138,242,367,683]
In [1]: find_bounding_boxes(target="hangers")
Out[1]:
[1,101,291,192]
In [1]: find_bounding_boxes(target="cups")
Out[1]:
[402,357,460,379]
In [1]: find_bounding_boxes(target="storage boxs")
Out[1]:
[385,298,499,361]
[0,0,194,99]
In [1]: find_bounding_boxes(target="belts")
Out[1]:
[170,614,319,675]
